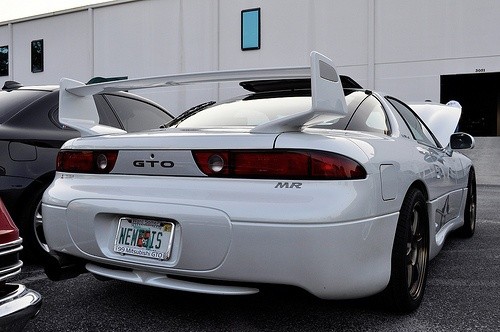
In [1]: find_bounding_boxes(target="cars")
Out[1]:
[0,81,180,265]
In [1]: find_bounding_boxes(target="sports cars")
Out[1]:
[41,51,478,312]
